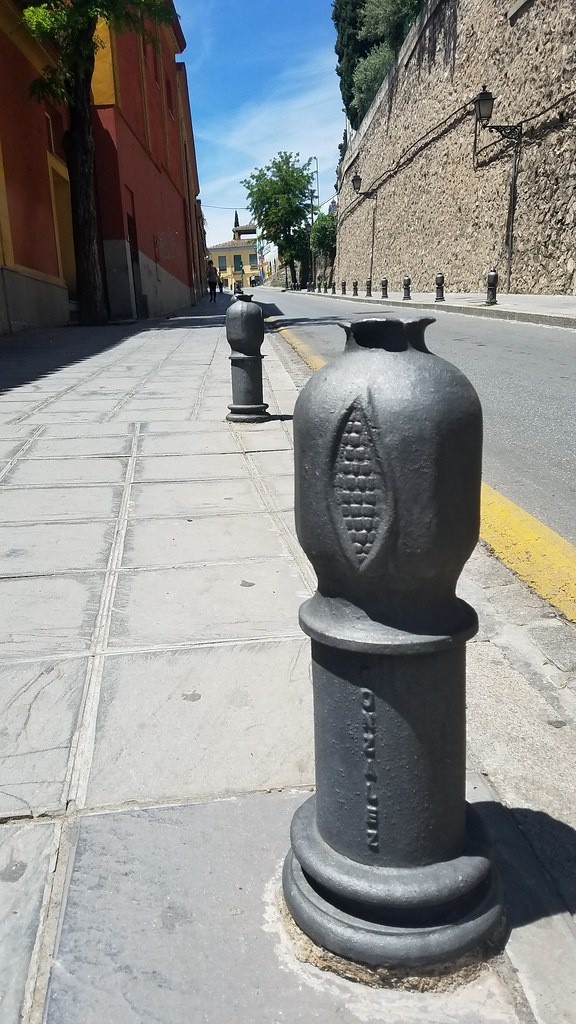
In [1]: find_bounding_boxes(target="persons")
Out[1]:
[206,259,221,304]
[250,274,257,287]
[216,272,225,295]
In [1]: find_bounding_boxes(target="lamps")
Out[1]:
[351,171,377,200]
[472,84,523,140]
[331,198,339,208]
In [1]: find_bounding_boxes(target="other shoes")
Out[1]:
[214,301,216,303]
[210,298,213,302]
